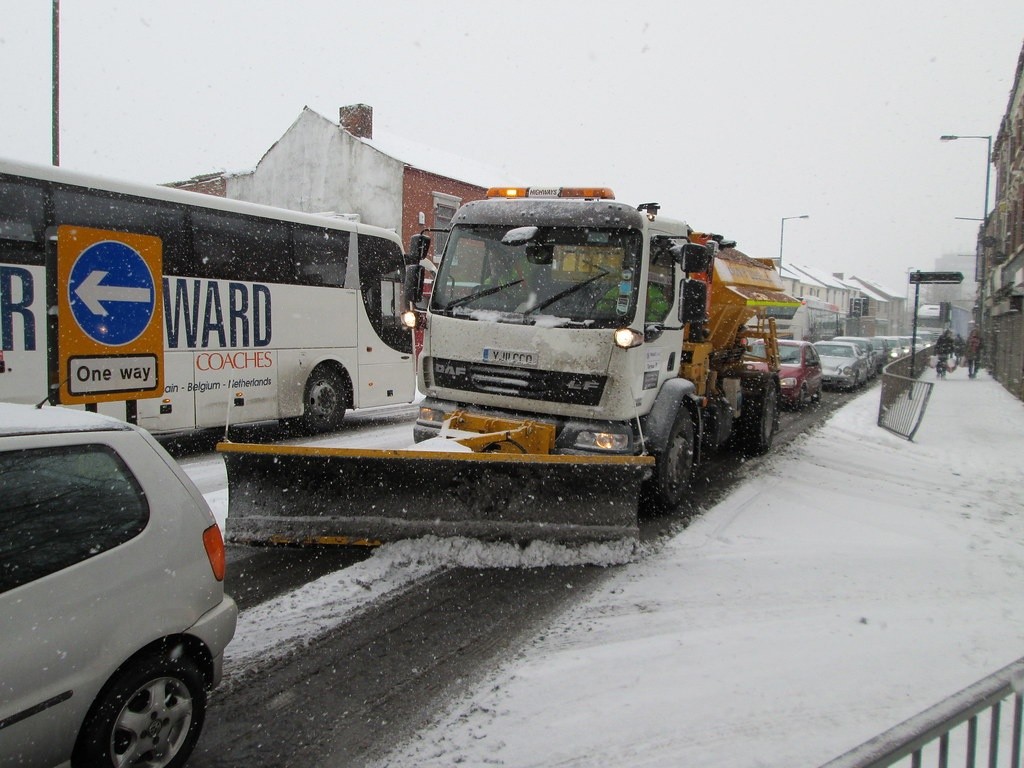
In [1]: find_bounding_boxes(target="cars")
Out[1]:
[0,400,240,768]
[749,335,921,410]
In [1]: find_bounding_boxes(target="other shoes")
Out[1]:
[969,374,977,378]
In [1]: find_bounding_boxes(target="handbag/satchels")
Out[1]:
[946,358,956,372]
[929,355,938,368]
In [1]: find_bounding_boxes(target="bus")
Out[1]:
[0,157,427,461]
[743,295,841,343]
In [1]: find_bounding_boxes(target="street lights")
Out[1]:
[779,215,808,280]
[940,135,993,366]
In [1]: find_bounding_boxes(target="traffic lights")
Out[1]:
[849,297,869,318]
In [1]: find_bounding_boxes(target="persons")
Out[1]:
[933,329,985,380]
[596,256,669,322]
[484,246,527,298]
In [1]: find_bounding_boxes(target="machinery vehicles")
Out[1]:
[215,187,801,546]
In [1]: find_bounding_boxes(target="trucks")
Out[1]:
[913,304,953,348]
